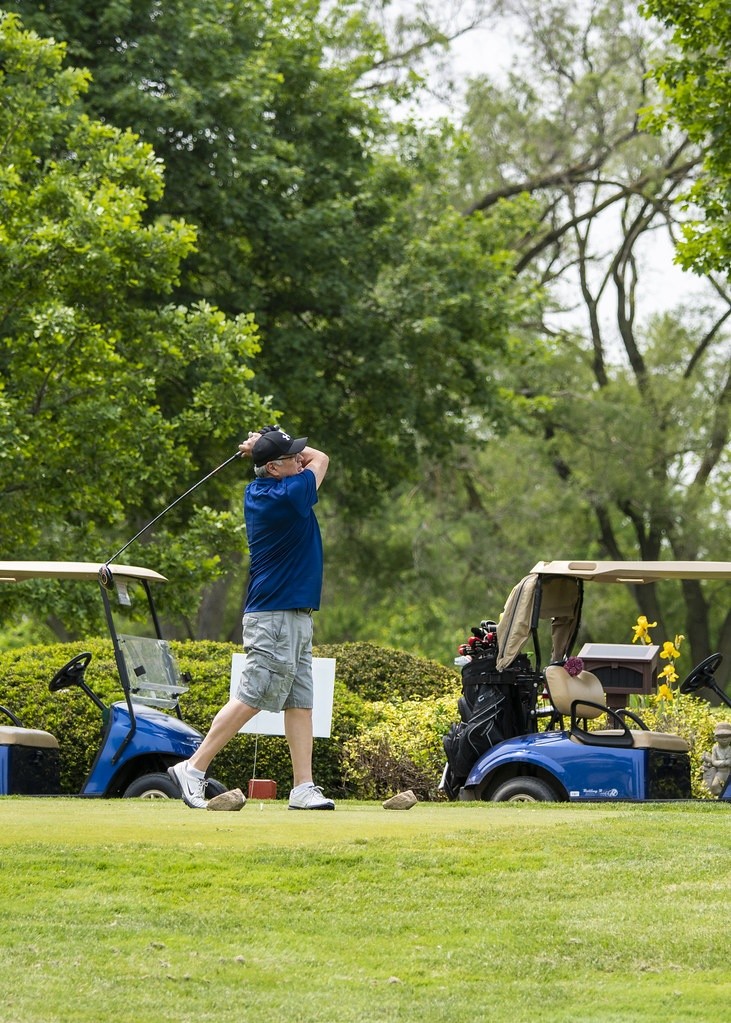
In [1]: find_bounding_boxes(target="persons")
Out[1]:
[710,723,731,796]
[164,429,336,809]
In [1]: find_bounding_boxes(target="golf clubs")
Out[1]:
[98,424,279,590]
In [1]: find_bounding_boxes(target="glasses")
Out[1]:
[277,454,298,461]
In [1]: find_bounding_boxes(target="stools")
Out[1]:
[0,705,59,749]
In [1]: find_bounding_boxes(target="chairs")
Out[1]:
[543,665,689,753]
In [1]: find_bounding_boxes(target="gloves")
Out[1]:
[258,425,281,436]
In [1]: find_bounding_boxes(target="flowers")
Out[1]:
[565,656,584,677]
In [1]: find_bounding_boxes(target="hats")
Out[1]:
[252,431,309,468]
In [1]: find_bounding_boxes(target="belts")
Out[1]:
[294,607,313,616]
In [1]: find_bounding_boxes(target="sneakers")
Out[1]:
[288,785,334,810]
[167,761,208,808]
